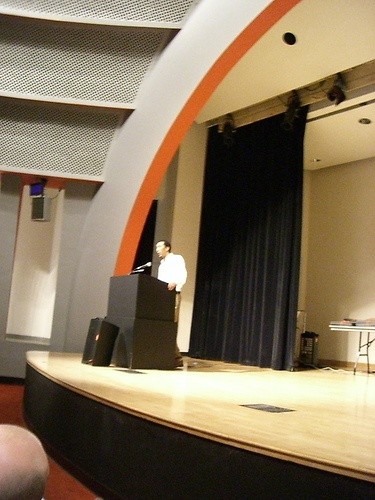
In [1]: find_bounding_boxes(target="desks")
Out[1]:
[328,325,375,375]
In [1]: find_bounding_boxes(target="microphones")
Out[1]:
[135,261,153,270]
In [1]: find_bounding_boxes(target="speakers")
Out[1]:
[31,197,50,222]
[81,318,120,367]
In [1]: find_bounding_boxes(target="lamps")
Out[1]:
[285,90,303,122]
[219,112,236,138]
[326,72,346,106]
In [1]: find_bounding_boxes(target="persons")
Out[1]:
[2,424,52,500]
[155,239,188,368]
